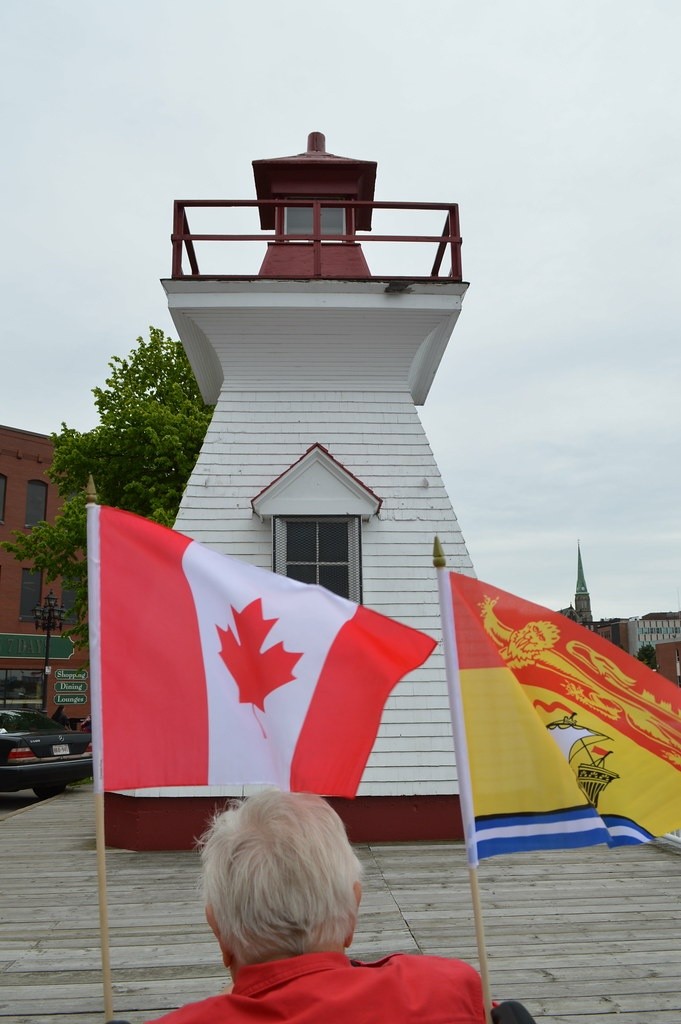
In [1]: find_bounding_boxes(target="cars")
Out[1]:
[0,708,94,800]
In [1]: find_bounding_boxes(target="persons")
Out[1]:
[52,705,70,730]
[81,715,91,732]
[142,789,499,1024]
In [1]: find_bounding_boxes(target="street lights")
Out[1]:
[30,588,66,718]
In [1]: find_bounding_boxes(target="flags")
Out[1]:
[439,568,681,864]
[87,505,438,798]
